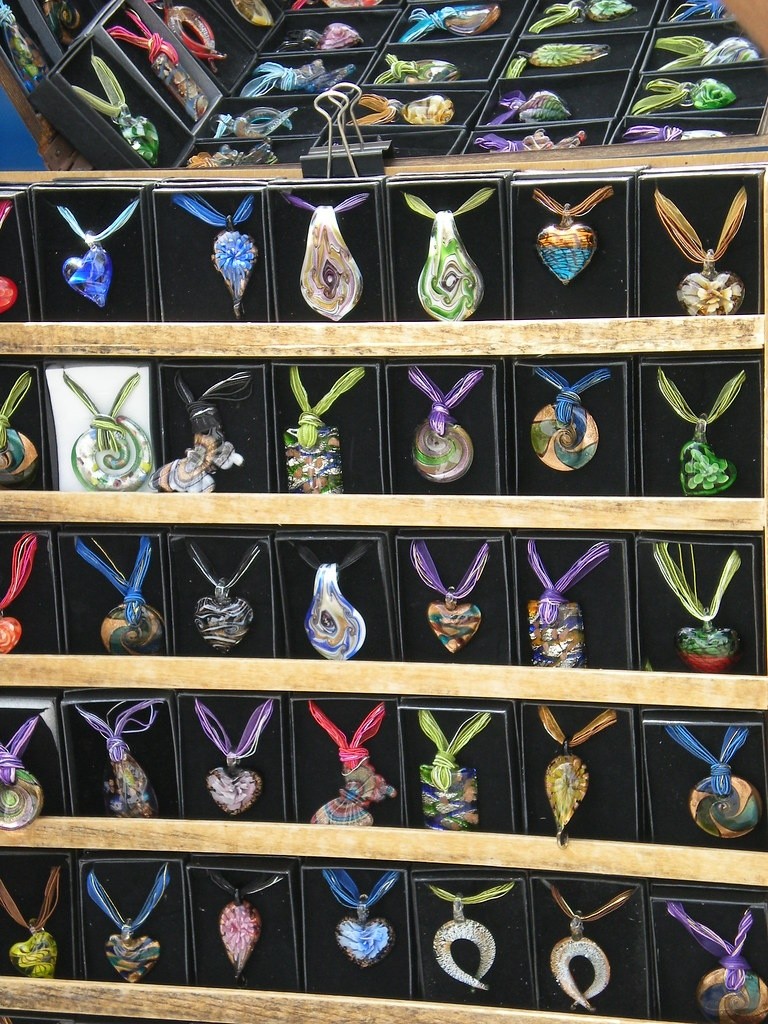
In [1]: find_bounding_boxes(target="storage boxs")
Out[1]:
[0,0,768,1024]
